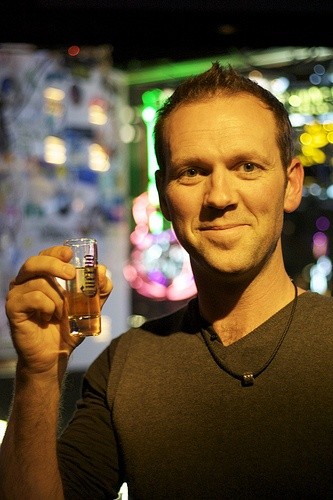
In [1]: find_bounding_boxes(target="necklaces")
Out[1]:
[194,277,298,385]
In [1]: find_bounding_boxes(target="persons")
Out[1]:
[0,55,333,500]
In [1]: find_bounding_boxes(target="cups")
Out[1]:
[63,238,102,336]
